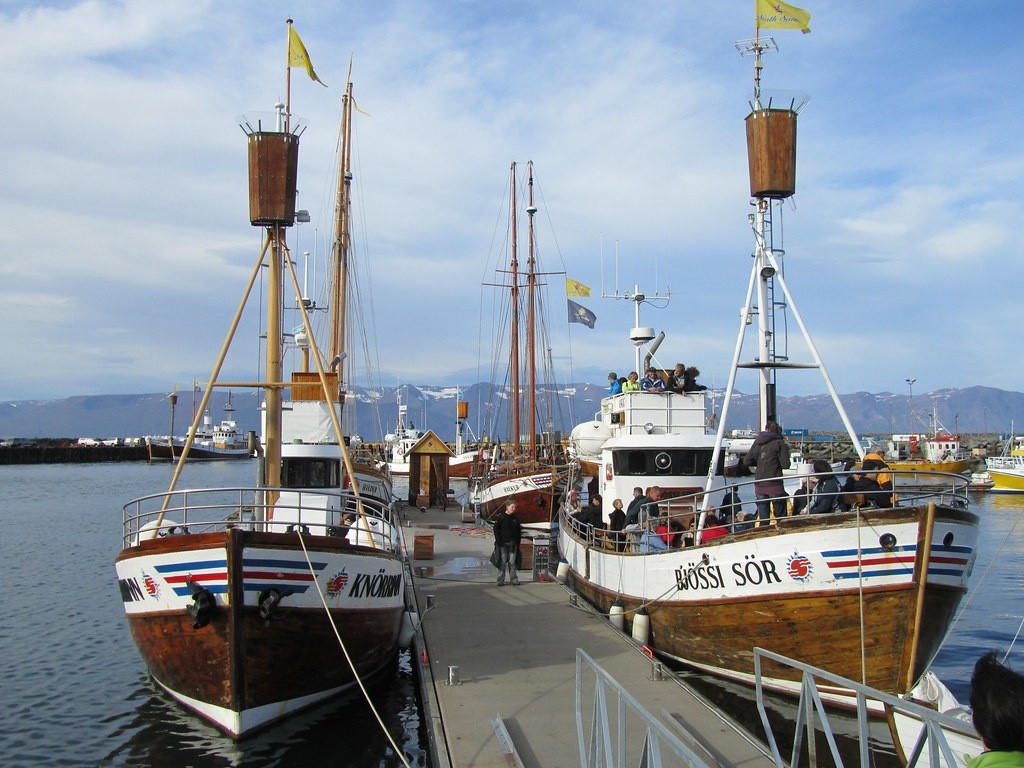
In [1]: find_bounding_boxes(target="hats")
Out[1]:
[850,467,862,472]
[802,476,819,483]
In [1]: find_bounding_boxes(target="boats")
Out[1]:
[854,420,1024,493]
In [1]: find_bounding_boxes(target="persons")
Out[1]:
[742,421,790,527]
[556,484,604,541]
[790,446,901,517]
[587,470,599,504]
[493,499,522,585]
[607,485,701,553]
[606,363,708,397]
[700,482,794,545]
[961,649,1024,768]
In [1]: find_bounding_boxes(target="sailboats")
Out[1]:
[447,161,579,534]
[557,0,980,724]
[114,13,406,744]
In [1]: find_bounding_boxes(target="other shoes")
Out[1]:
[510,579,520,585]
[497,582,504,586]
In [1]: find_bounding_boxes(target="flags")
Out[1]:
[286,25,329,88]
[565,279,591,297]
[566,299,598,329]
[755,0,812,32]
